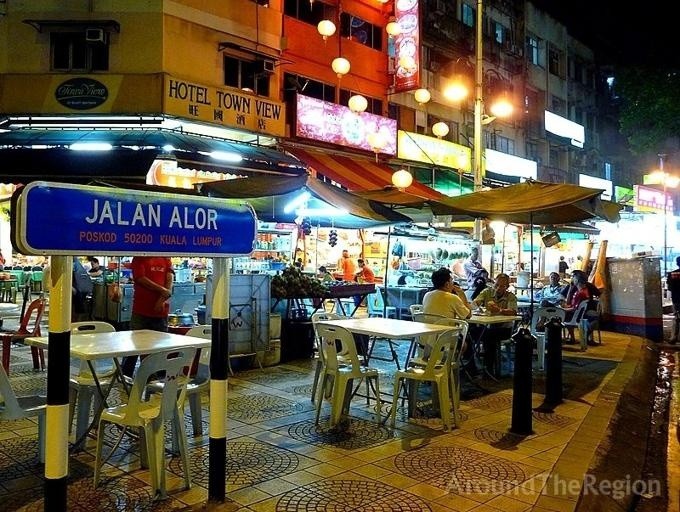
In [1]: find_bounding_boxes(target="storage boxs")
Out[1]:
[269,313,282,339]
[252,339,281,368]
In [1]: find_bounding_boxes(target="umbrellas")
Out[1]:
[199,172,416,264]
[433,176,627,321]
[343,184,441,318]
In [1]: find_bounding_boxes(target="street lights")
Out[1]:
[443,54,515,242]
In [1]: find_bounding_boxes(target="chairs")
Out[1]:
[140,324,215,456]
[93,345,198,501]
[0,265,122,464]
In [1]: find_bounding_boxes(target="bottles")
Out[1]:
[233,257,269,274]
[255,233,272,251]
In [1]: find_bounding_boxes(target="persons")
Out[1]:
[534,255,600,346]
[467,273,518,382]
[83,255,95,272]
[319,266,332,277]
[88,258,104,277]
[354,259,375,307]
[422,268,474,365]
[464,248,492,290]
[510,263,524,276]
[121,257,173,384]
[667,256,680,345]
[338,250,357,282]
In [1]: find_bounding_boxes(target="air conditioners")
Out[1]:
[86,28,107,45]
[255,59,275,76]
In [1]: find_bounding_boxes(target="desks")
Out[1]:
[24,329,214,474]
